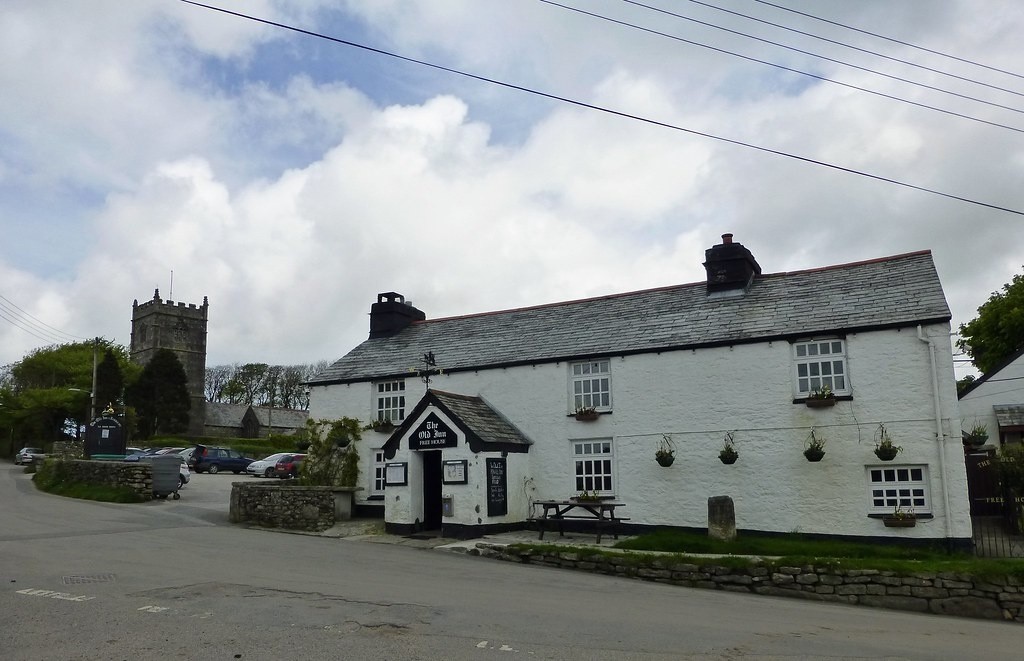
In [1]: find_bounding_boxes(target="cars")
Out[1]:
[124,444,239,490]
[14,447,44,465]
[246,452,301,479]
[276,452,308,479]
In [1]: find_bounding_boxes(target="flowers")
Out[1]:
[576,404,599,413]
[890,507,915,522]
[374,419,394,425]
[809,384,834,399]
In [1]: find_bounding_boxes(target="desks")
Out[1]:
[533,501,626,544]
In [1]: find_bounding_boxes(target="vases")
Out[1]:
[884,519,915,527]
[576,414,598,421]
[806,398,835,407]
[375,426,394,432]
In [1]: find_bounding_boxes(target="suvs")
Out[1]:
[193,443,257,475]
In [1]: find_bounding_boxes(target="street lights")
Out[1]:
[67,387,97,421]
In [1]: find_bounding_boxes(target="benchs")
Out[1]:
[526,515,630,528]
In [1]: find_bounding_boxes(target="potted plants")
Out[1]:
[655,446,675,467]
[966,417,988,446]
[718,444,739,464]
[577,489,602,503]
[803,436,827,462]
[874,437,903,461]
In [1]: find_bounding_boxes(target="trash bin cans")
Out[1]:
[138,454,183,500]
